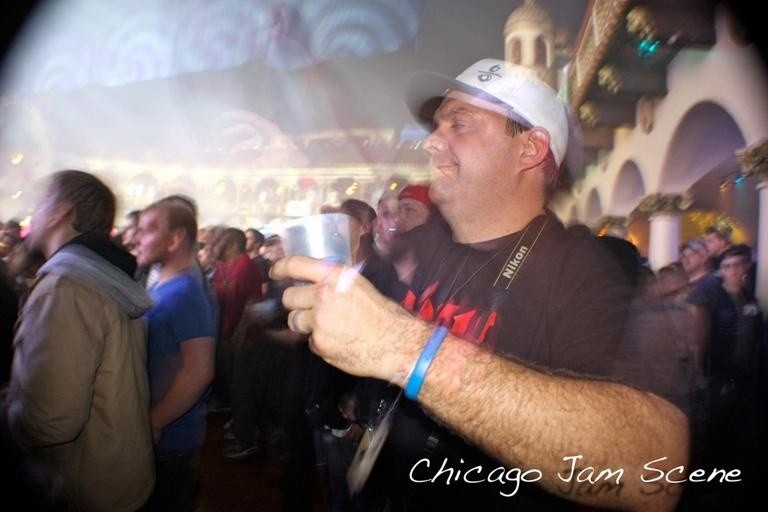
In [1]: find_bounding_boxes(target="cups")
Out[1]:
[276,213,355,288]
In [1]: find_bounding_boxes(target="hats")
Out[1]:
[320,200,377,228]
[403,57,569,170]
[397,184,429,205]
[680,240,710,260]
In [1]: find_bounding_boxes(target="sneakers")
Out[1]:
[223,442,256,458]
[208,402,232,413]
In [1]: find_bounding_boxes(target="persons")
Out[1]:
[2,57,768,511]
